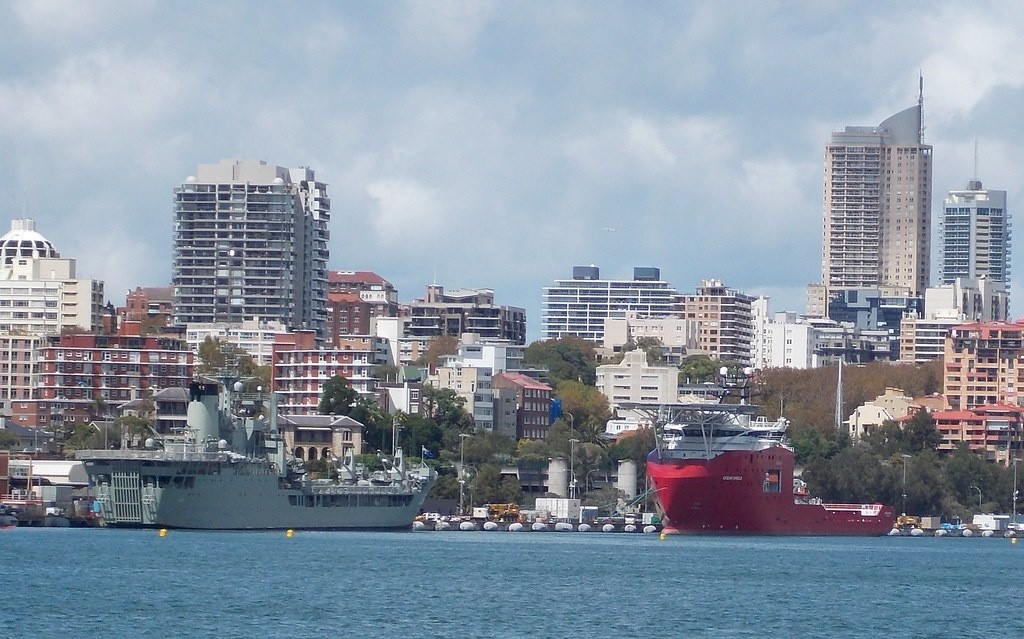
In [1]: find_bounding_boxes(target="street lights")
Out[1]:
[568,438,580,499]
[458,432,470,515]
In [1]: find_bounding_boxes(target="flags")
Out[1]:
[423,447,434,458]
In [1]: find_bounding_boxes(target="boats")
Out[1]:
[643,363,896,539]
[74,327,440,532]
